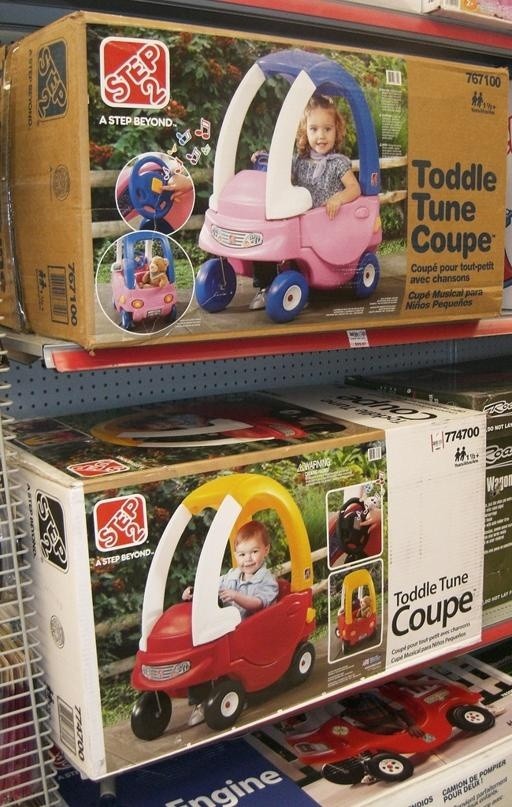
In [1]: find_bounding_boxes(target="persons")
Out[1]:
[336,692,425,783]
[182,520,278,729]
[161,170,191,201]
[247,93,360,312]
[361,506,383,534]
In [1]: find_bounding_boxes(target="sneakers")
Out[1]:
[247,286,270,311]
[359,773,382,786]
[187,699,206,727]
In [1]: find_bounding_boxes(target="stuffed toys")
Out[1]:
[139,254,170,288]
[355,595,372,619]
